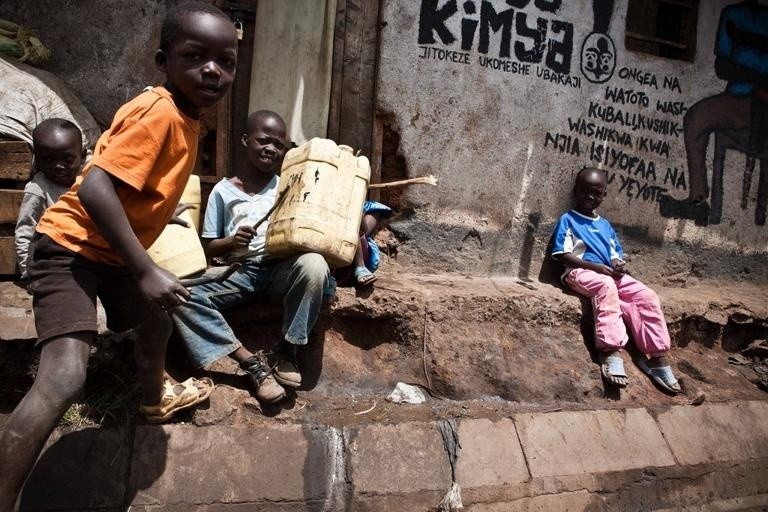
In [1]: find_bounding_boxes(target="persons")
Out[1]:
[165,110,337,404]
[551,168,681,393]
[0,1,239,512]
[661,0,768,227]
[15,119,89,295]
[332,200,392,286]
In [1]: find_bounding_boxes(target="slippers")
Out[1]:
[637,353,684,396]
[352,263,377,291]
[598,351,629,389]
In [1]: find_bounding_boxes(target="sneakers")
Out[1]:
[269,336,305,390]
[238,354,287,405]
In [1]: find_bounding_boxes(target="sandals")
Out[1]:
[138,375,215,425]
